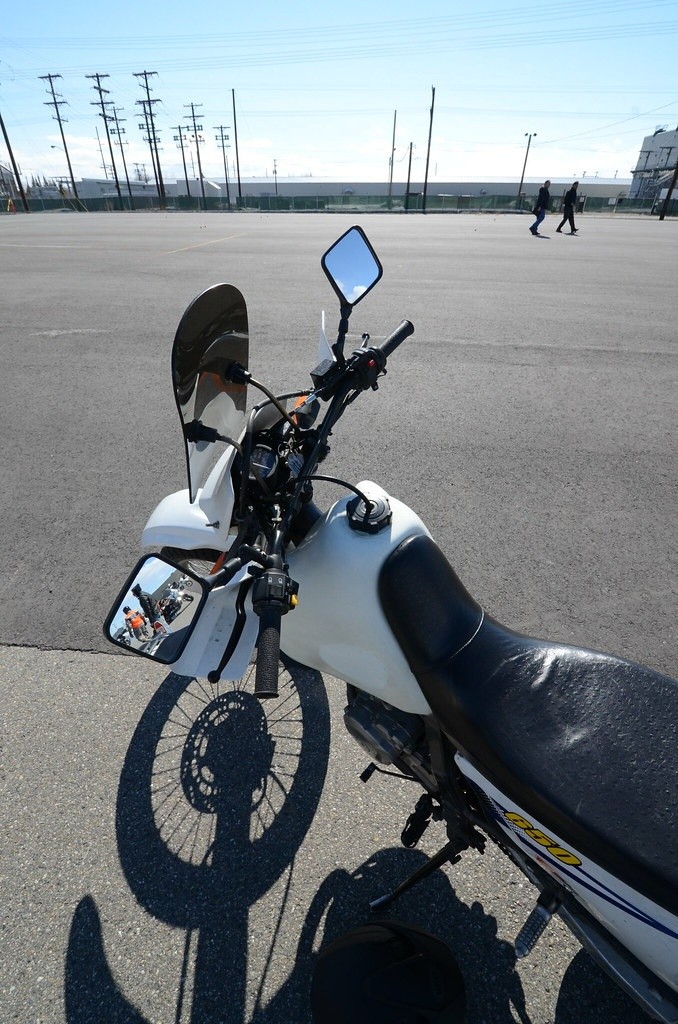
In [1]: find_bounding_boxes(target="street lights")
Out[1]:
[515,132,538,209]
[51,145,82,210]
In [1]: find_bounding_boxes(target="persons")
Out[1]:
[529,180,550,235]
[556,181,579,234]
[123,606,150,642]
[131,583,173,634]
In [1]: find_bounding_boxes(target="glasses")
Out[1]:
[133,593,135,596]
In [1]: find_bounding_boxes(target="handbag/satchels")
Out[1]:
[532,207,541,217]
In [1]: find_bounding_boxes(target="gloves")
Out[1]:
[130,632,134,638]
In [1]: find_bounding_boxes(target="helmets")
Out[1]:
[123,606,131,614]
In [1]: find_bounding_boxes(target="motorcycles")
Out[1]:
[103,226,678,1024]
[159,576,193,625]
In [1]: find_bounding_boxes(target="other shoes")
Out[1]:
[556,228,562,233]
[571,229,578,234]
[529,227,540,235]
